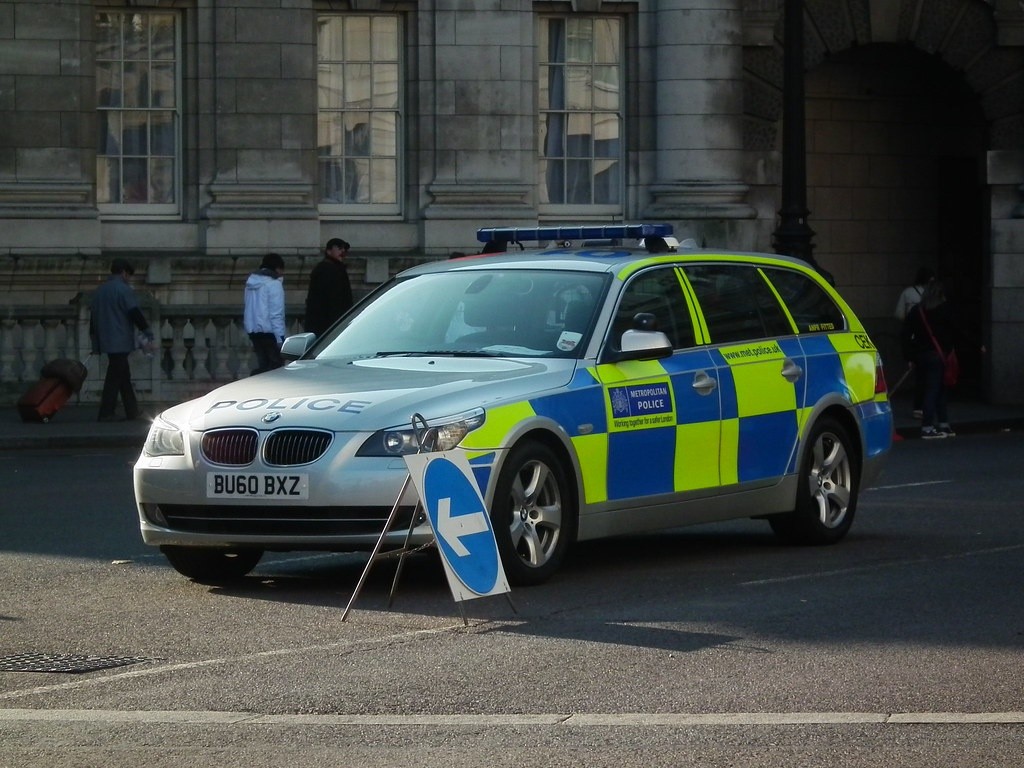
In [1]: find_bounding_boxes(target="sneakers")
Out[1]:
[912,409,924,419]
[934,422,956,437]
[920,426,948,440]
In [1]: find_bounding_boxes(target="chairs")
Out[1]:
[452,276,557,350]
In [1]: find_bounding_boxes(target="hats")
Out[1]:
[327,238,350,250]
[112,258,134,271]
[259,253,285,269]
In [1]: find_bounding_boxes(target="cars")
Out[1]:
[133,223,895,588]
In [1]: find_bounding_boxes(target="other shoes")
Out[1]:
[98,413,126,422]
[893,431,904,441]
[127,407,144,420]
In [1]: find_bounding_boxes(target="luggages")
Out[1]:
[18,350,101,424]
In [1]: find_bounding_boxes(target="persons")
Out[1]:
[243,253,286,372]
[304,238,354,340]
[896,263,988,439]
[89,258,154,420]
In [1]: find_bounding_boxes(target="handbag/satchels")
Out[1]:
[943,349,960,385]
[40,358,87,393]
